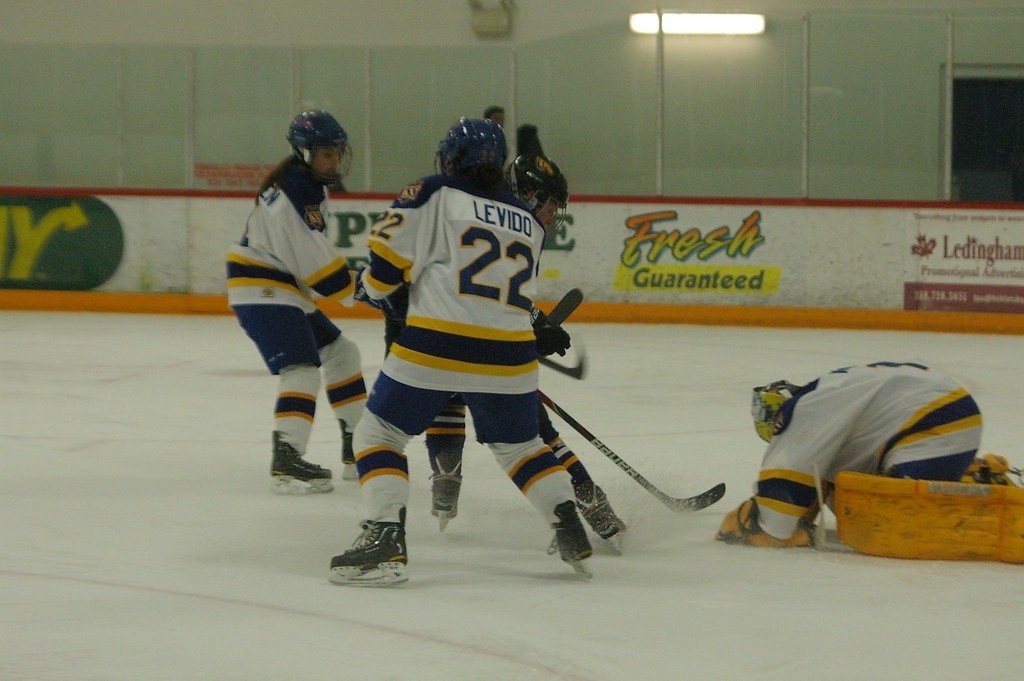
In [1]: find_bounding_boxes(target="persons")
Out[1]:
[331,117,591,569]
[386,106,625,543]
[717,361,1009,546]
[227,111,369,495]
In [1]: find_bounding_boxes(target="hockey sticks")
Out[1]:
[343,261,725,515]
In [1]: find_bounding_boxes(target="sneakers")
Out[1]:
[428,450,463,532]
[547,499,596,583]
[270,429,335,496]
[336,418,359,481]
[571,476,627,556]
[328,505,410,589]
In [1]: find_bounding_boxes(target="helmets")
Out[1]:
[751,385,790,443]
[286,109,352,189]
[434,116,508,180]
[506,152,567,239]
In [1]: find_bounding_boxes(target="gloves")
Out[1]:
[354,267,396,316]
[530,306,571,357]
